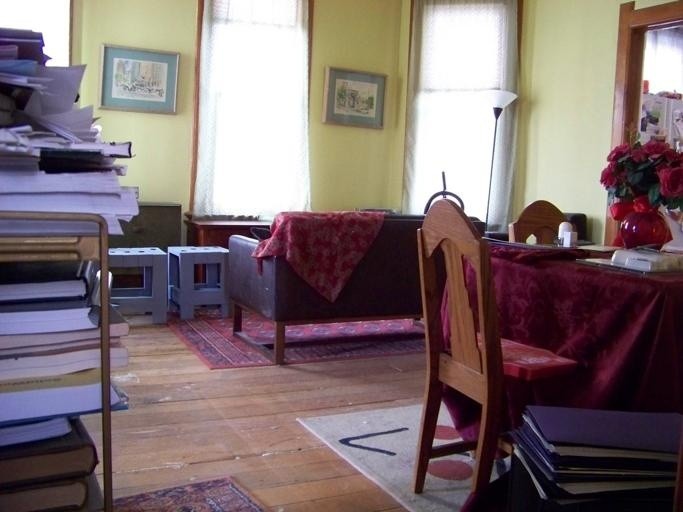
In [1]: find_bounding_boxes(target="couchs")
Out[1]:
[226,214,487,365]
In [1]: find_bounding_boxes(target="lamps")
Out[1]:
[480,89,515,230]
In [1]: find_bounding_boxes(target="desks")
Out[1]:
[438,246,683,459]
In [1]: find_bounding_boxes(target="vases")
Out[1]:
[618,204,672,248]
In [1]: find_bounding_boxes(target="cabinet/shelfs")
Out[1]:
[2,25,140,502]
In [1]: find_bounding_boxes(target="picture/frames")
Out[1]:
[323,67,385,128]
[100,45,178,116]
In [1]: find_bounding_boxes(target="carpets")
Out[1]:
[169,298,430,370]
[296,401,512,512]
[103,474,273,512]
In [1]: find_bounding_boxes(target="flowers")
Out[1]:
[599,140,682,209]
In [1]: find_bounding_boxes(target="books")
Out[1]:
[0,480,89,512]
[0,341,131,379]
[0,337,122,357]
[24,138,134,159]
[0,298,100,334]
[0,418,71,447]
[0,418,99,487]
[0,212,124,239]
[0,304,131,350]
[0,186,140,223]
[0,257,97,301]
[0,170,121,199]
[0,367,121,422]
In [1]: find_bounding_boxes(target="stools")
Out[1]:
[107,248,168,325]
[168,245,229,320]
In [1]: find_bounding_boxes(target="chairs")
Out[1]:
[510,202,579,243]
[413,201,573,495]
